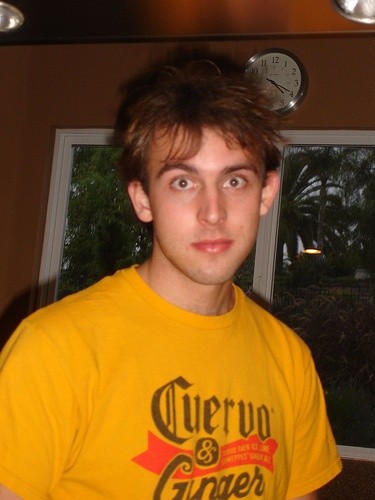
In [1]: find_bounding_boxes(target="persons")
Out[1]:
[0,48,345,500]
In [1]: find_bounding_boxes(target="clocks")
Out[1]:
[240,47,308,117]
